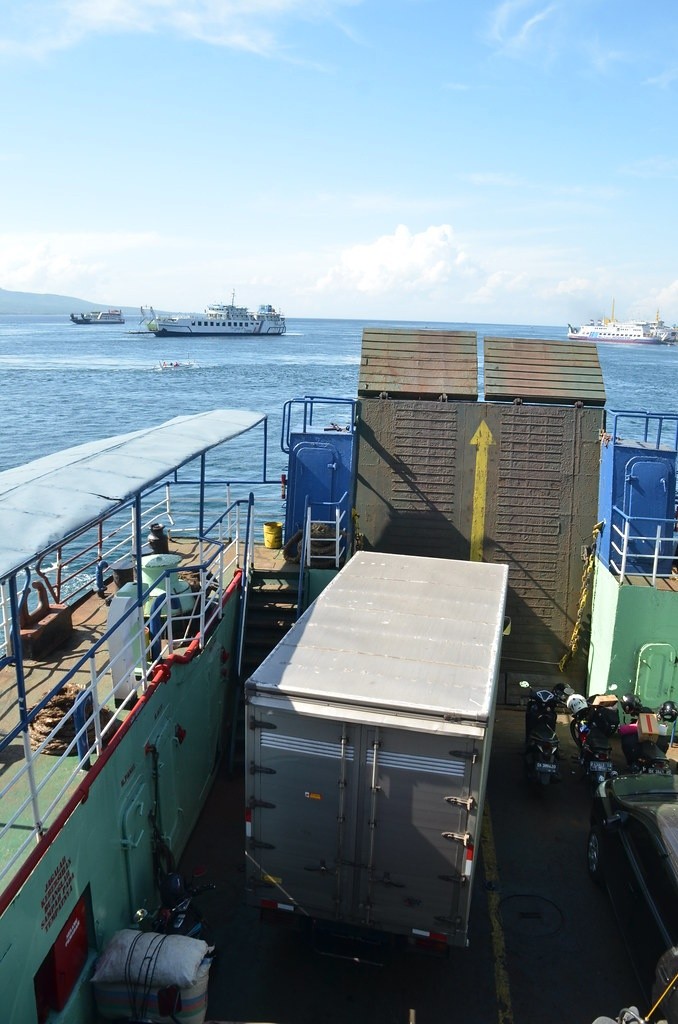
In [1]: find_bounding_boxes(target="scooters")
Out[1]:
[622,706,675,775]
[519,681,565,788]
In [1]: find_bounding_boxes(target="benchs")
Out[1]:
[17,581,72,659]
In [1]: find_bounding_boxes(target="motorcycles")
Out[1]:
[556,683,617,790]
[133,864,215,1023]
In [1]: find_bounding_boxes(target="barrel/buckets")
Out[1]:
[263,522,283,548]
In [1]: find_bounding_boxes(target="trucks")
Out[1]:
[243,549,512,950]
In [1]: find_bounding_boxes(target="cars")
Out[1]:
[587,774,678,1024]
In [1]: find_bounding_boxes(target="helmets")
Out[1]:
[551,682,572,706]
[619,693,642,715]
[159,869,185,905]
[659,701,678,722]
[566,694,588,715]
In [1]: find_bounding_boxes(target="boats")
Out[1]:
[138,290,286,337]
[159,362,193,370]
[70,310,125,324]
[567,299,678,344]
